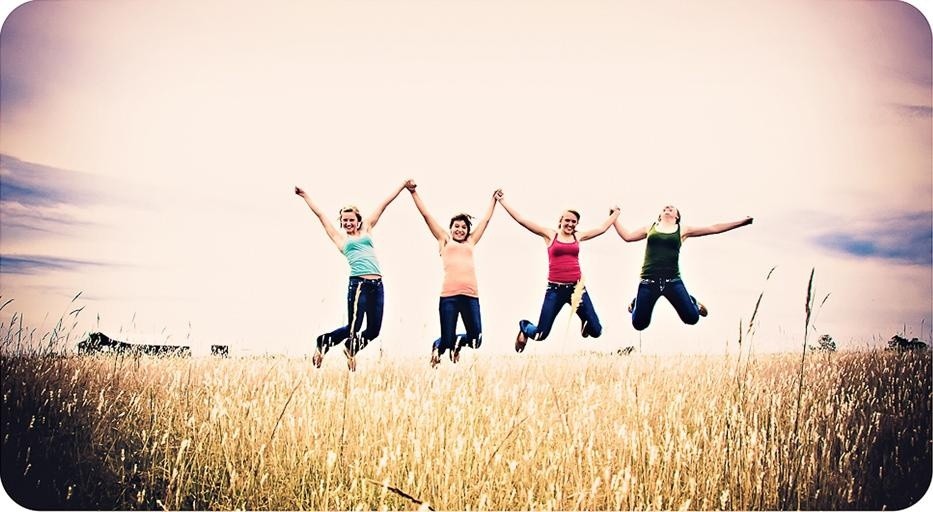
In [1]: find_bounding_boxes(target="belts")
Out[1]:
[350,276,381,283]
[643,277,680,283]
[548,282,575,289]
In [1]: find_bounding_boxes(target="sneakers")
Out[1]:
[580,320,590,337]
[311,347,323,368]
[514,330,528,353]
[429,346,441,369]
[449,348,460,363]
[342,349,356,371]
[696,303,707,318]
[627,298,635,313]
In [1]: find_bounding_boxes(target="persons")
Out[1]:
[407,179,503,368]
[495,190,621,353]
[610,205,754,330]
[295,182,410,370]
